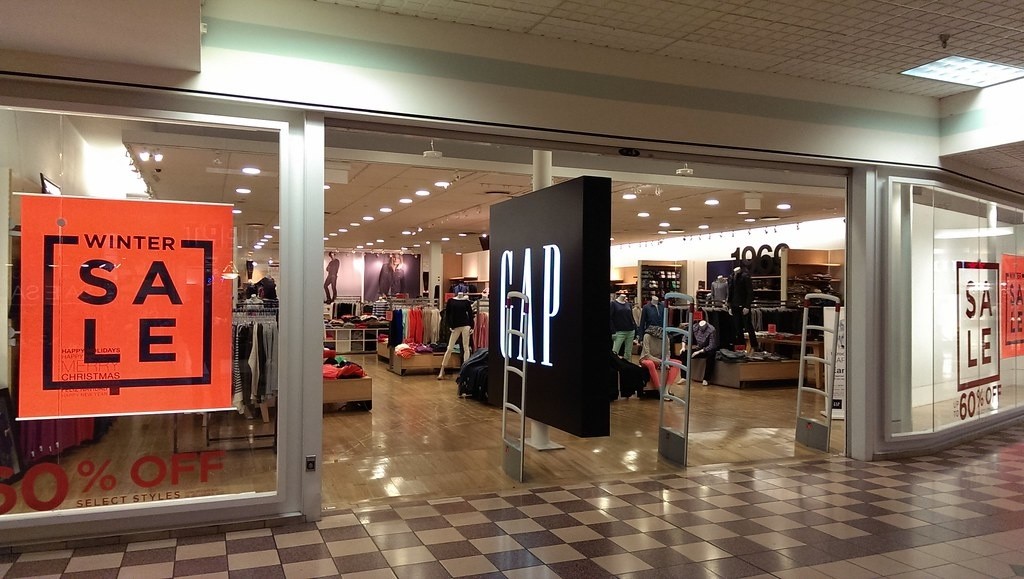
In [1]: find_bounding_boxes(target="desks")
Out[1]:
[323,375,374,411]
[743,334,824,389]
[393,351,461,376]
[376,339,391,364]
[688,357,800,388]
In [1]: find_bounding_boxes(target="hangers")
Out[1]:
[334,296,436,310]
[232,299,279,326]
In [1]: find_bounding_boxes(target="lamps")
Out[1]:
[720,232,723,238]
[151,174,161,183]
[796,223,800,231]
[657,239,663,245]
[708,233,711,239]
[423,140,442,160]
[774,226,778,233]
[140,148,164,163]
[412,206,482,237]
[698,234,701,240]
[683,237,686,242]
[732,230,734,237]
[747,228,751,235]
[221,261,241,280]
[689,236,693,242]
[764,226,768,234]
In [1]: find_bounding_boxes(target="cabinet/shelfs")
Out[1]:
[610,246,845,308]
[323,328,390,354]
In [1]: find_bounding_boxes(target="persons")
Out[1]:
[373,298,389,318]
[379,253,409,297]
[710,267,761,352]
[610,294,682,401]
[246,261,254,282]
[437,293,489,379]
[677,320,717,385]
[324,251,340,304]
[416,295,424,303]
[255,274,277,300]
